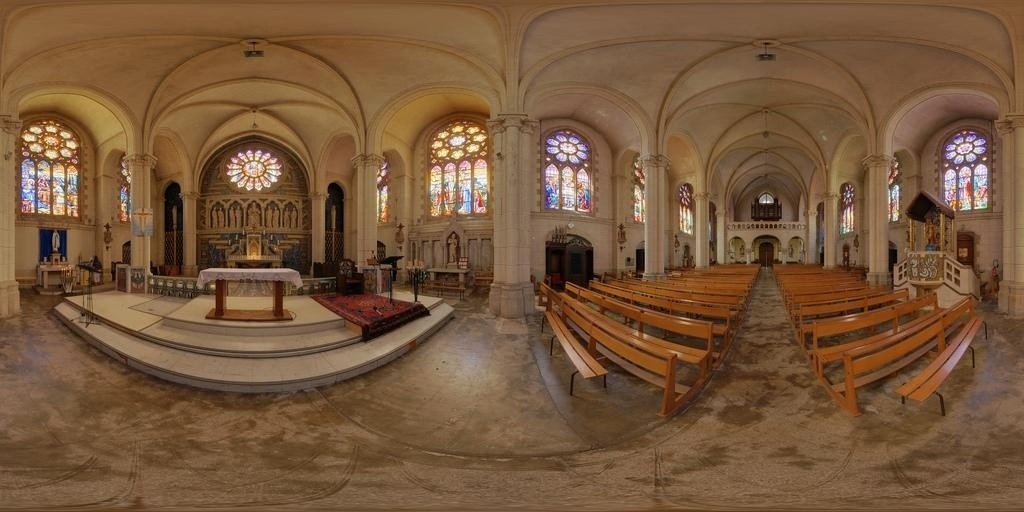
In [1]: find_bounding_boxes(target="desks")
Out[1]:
[194,268,303,321]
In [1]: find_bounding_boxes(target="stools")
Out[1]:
[318,281,329,296]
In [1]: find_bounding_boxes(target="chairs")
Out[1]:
[336,259,364,295]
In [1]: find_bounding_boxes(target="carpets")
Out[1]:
[309,291,431,342]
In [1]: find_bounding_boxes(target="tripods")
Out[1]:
[358,266,384,317]
[70,269,100,328]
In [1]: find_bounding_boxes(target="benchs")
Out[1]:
[434,286,466,300]
[473,276,493,291]
[530,261,992,421]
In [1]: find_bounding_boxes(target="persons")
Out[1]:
[446,232,458,264]
